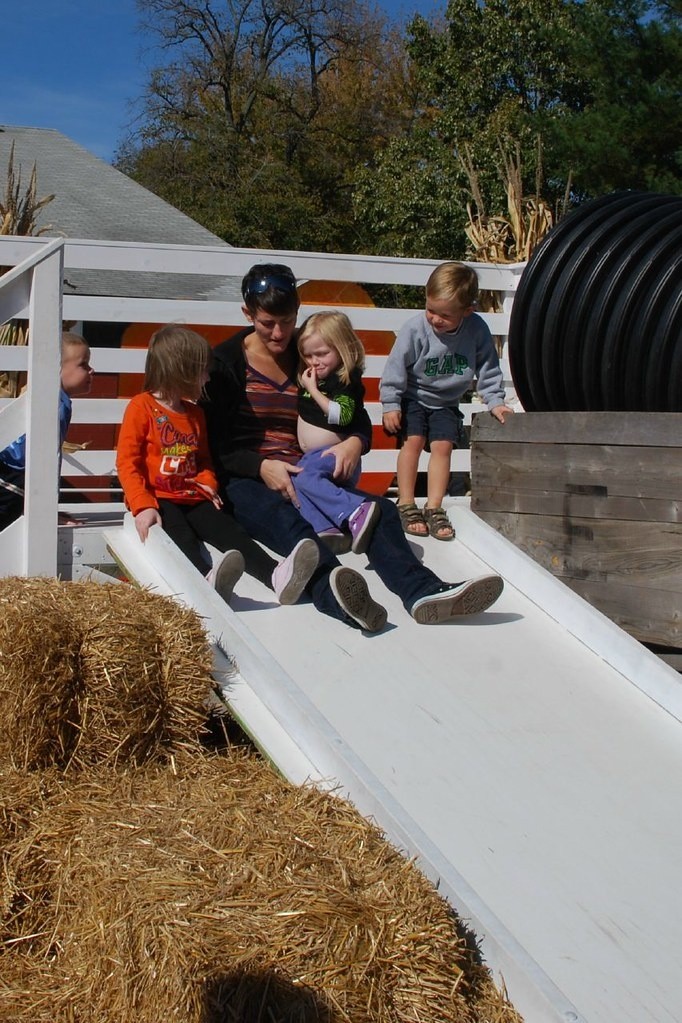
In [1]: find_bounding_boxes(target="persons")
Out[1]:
[378,261,514,541]
[116,264,504,633]
[0,331,94,532]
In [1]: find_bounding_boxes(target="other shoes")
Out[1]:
[205,549,245,604]
[317,527,352,552]
[271,538,320,605]
[348,501,381,554]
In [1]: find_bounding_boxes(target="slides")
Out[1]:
[111,502,682,1023]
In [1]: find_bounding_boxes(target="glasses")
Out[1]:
[244,275,296,298]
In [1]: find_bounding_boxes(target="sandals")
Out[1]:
[421,501,456,540]
[395,497,429,537]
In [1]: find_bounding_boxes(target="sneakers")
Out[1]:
[410,573,504,625]
[328,566,388,633]
